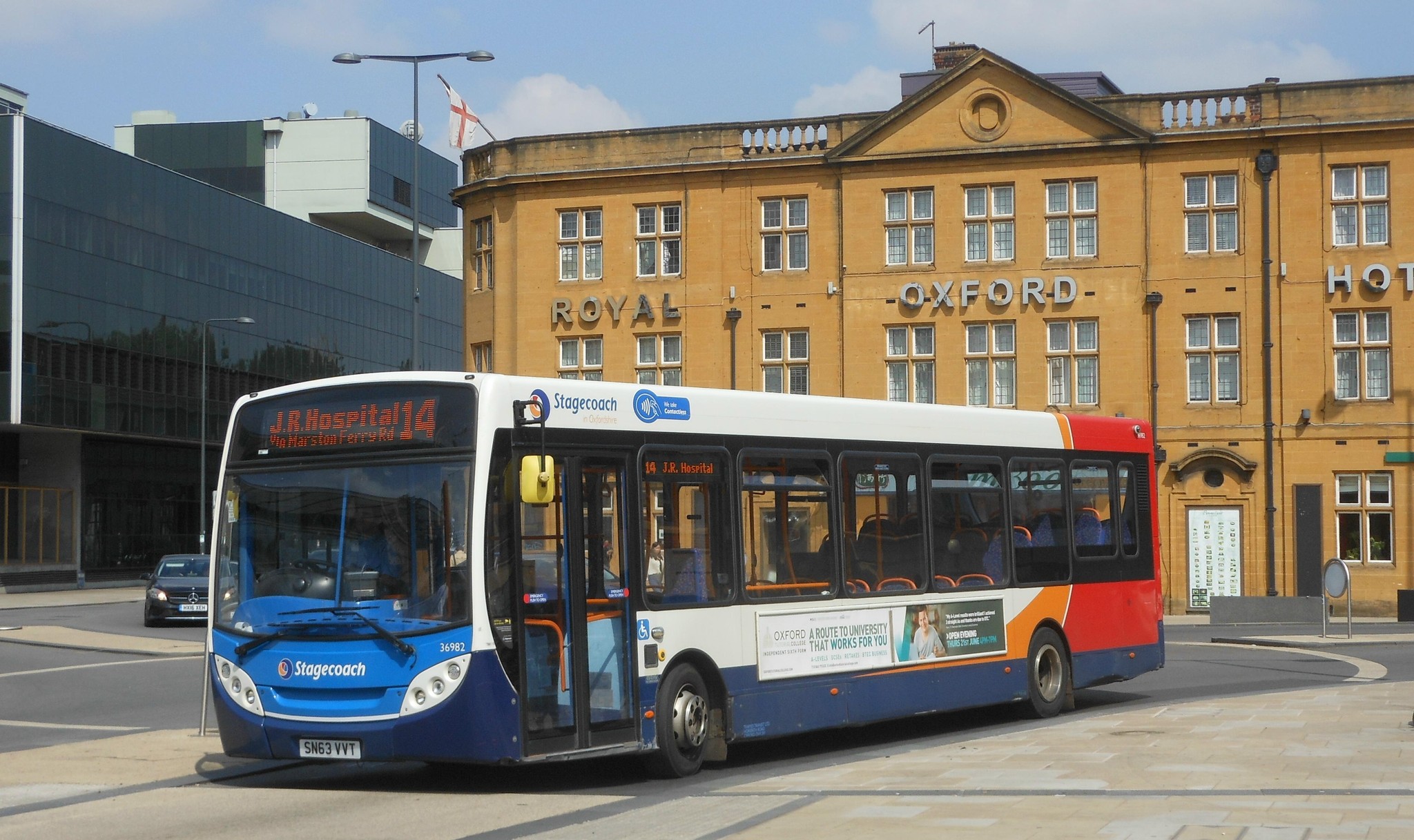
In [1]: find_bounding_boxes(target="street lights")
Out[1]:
[333,50,494,373]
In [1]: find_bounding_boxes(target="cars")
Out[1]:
[141,554,212,627]
[522,551,620,590]
[310,549,377,596]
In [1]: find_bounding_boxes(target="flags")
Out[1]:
[438,76,478,151]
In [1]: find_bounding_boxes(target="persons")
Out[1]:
[323,508,400,593]
[646,541,667,593]
[603,543,615,570]
[913,605,946,660]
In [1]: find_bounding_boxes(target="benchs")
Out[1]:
[819,508,1130,595]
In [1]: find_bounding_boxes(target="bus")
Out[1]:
[207,370,1164,778]
